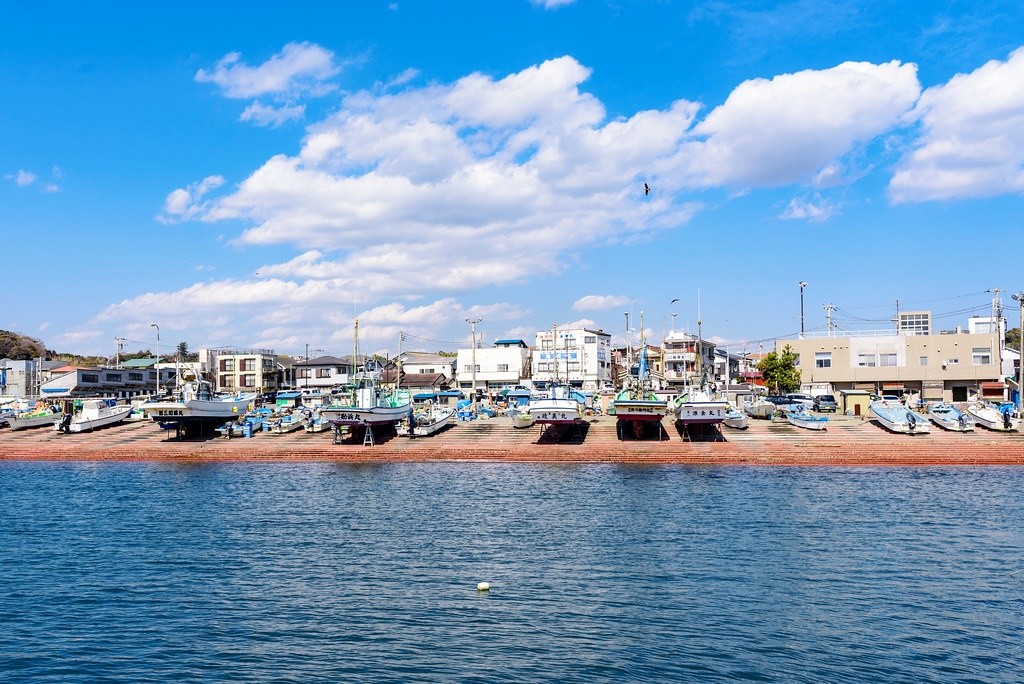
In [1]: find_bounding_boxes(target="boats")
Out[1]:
[871,401,931,435]
[4,403,55,429]
[967,402,1022,431]
[507,405,533,426]
[139,378,257,436]
[724,406,749,429]
[786,411,828,430]
[529,360,586,436]
[395,406,455,436]
[613,384,667,440]
[673,382,728,440]
[927,401,974,432]
[743,396,775,417]
[313,319,411,440]
[52,398,134,432]
[213,403,333,436]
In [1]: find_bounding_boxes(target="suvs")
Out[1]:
[786,393,815,409]
[813,395,836,412]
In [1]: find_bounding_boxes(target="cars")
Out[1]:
[764,396,803,410]
[262,392,276,403]
[876,395,902,404]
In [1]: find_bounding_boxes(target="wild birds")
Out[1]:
[644,183,650,196]
[671,299,680,304]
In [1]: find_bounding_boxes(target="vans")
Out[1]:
[302,389,320,394]
[277,389,301,395]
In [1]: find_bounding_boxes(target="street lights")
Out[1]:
[663,313,677,377]
[560,333,572,383]
[624,312,629,381]
[465,317,482,404]
[150,323,159,395]
